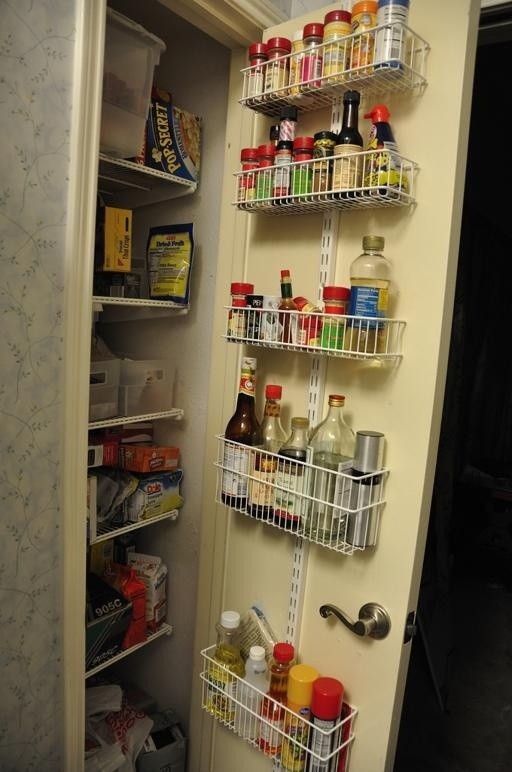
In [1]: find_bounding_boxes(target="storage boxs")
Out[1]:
[137,726,187,770]
[86,352,122,424]
[114,352,178,419]
[98,6,168,156]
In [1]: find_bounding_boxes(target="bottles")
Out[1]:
[221,357,385,548]
[238,90,407,202]
[229,234,394,357]
[209,609,345,771]
[247,0,410,101]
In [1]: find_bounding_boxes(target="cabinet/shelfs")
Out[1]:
[86,147,205,771]
[196,23,432,771]
